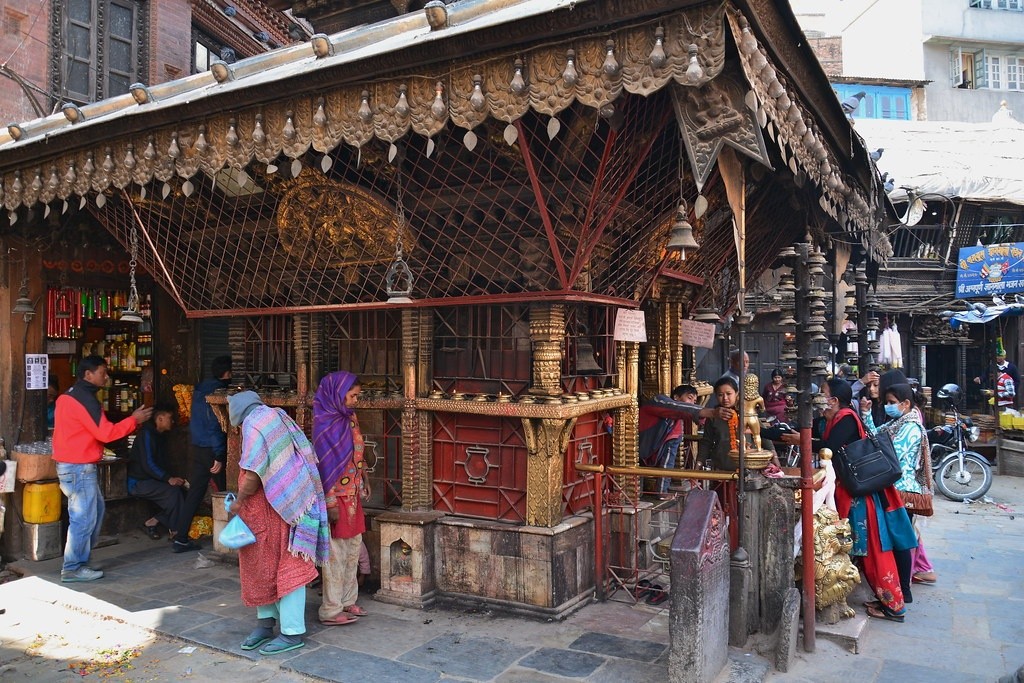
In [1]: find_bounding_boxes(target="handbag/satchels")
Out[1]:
[219,492,257,549]
[831,414,902,497]
[898,491,933,517]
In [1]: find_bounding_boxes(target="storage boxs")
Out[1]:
[10,450,58,484]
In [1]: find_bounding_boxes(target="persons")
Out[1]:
[52,354,156,581]
[974,348,1021,413]
[129,82,152,105]
[735,374,765,453]
[637,349,933,621]
[424,1,448,30]
[225,391,319,655]
[311,34,336,59]
[129,403,185,538]
[172,357,231,552]
[61,104,86,123]
[211,60,235,84]
[311,369,369,624]
[7,123,28,140]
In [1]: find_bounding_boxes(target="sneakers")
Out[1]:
[60,565,104,582]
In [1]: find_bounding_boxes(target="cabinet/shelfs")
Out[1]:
[37,266,158,442]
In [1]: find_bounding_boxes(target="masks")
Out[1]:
[884,401,906,419]
[812,393,835,414]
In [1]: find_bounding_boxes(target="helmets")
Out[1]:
[937,383,964,406]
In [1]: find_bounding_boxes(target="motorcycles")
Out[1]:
[925,399,993,503]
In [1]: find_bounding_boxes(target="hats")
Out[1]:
[227,391,264,428]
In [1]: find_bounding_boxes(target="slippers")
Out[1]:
[646,584,669,605]
[632,579,652,598]
[321,611,358,626]
[865,607,904,622]
[259,641,305,656]
[241,635,271,650]
[863,601,885,608]
[344,605,368,616]
[140,523,161,540]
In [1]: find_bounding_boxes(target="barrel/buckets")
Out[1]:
[22,478,63,524]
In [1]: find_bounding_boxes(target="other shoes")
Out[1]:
[912,572,937,583]
[172,541,202,553]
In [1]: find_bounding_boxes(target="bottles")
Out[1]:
[81,292,154,373]
[100,378,145,415]
[14,435,52,455]
